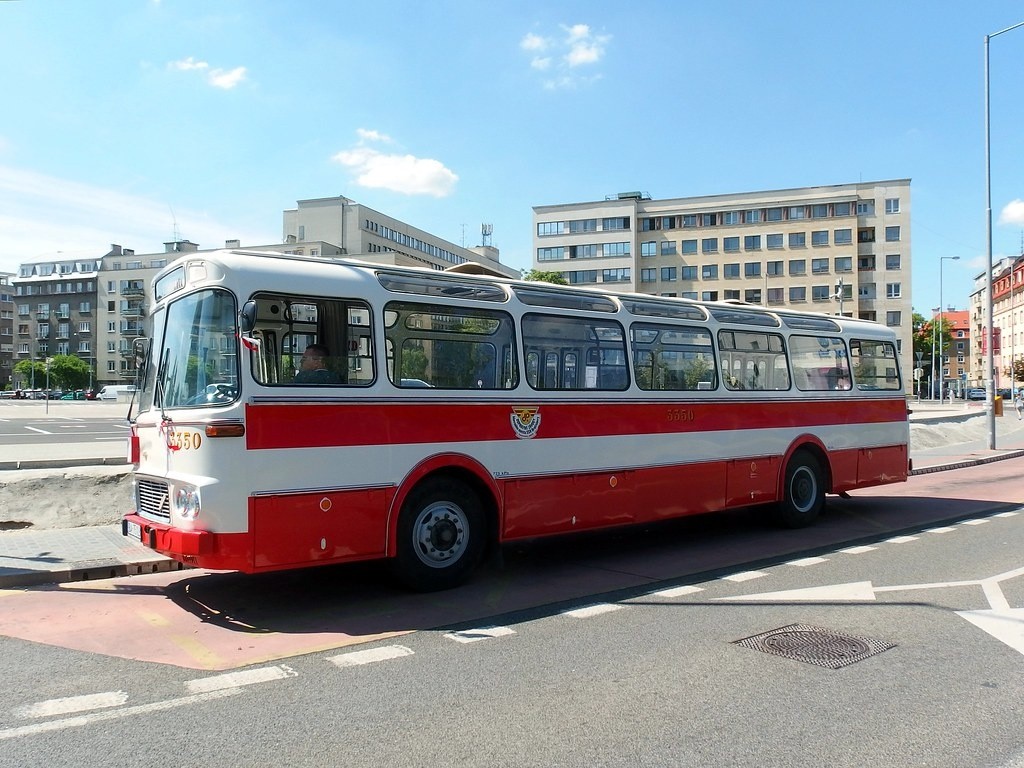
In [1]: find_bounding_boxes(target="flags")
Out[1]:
[235,333,258,351]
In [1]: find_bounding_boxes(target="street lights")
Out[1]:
[931,306,940,401]
[938,255,961,404]
[828,275,844,317]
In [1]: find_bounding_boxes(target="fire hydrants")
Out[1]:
[948,389,956,404]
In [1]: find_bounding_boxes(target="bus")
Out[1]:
[121,247,914,594]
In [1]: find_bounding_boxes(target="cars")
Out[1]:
[946,388,1019,401]
[0,388,95,401]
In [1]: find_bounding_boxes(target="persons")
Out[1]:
[292,344,342,384]
[826,368,849,390]
[475,346,495,390]
[949,389,955,405]
[1015,393,1023,420]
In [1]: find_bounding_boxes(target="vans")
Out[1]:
[95,384,136,400]
